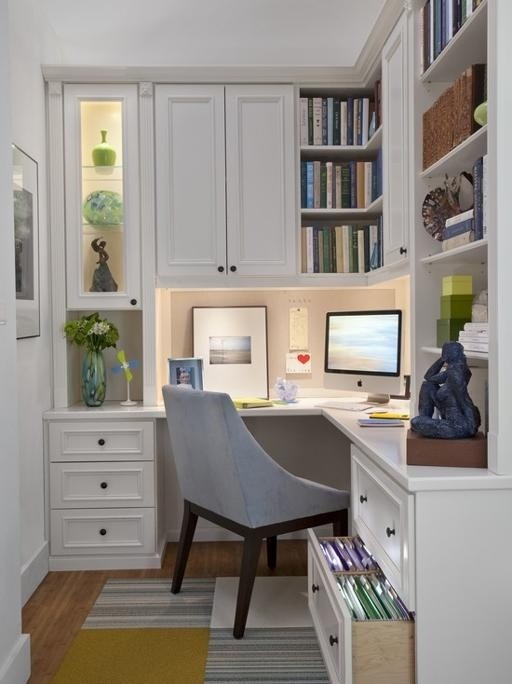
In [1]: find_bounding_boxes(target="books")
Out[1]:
[439,158,487,251]
[457,323,489,362]
[357,418,403,427]
[302,150,381,209]
[300,81,381,146]
[302,218,384,272]
[422,0,481,71]
[234,397,271,408]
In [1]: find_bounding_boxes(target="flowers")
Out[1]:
[62,312,120,351]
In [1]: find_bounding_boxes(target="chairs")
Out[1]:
[162,384,348,640]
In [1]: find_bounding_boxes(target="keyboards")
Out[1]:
[313,399,375,412]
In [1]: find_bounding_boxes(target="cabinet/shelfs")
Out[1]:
[382,11,411,278]
[411,1,500,473]
[299,64,384,286]
[306,446,512,684]
[44,418,164,570]
[153,83,299,291]
[62,80,144,313]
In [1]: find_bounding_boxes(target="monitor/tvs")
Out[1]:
[323,306,402,396]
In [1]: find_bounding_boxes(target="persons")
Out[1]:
[177,367,194,390]
[410,341,481,439]
[89,235,118,292]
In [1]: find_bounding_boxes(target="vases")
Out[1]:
[80,351,107,408]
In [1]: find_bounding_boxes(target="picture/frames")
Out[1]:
[12,145,41,340]
[167,356,203,393]
[191,305,269,400]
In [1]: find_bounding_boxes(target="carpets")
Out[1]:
[55,578,336,684]
